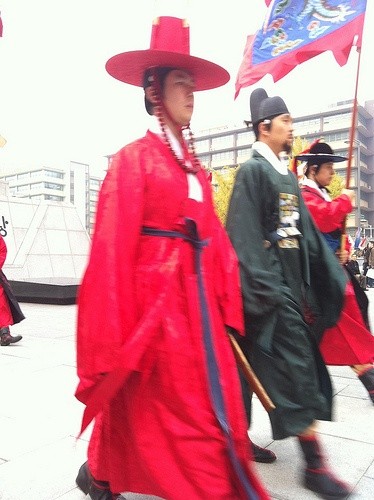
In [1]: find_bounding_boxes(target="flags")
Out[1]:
[234,0,368,99]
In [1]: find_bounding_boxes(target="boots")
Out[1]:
[0,325,23,346]
[298,435,349,499]
[246,432,277,463]
[358,366,374,404]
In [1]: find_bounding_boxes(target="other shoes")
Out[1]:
[76,460,128,500]
[363,288,368,291]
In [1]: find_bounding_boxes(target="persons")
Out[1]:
[0,235,25,346]
[347,254,368,291]
[292,141,374,407]
[226,88,349,500]
[361,241,374,288]
[74,67,272,500]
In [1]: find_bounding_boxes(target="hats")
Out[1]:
[294,142,349,164]
[249,88,289,126]
[105,16,230,91]
